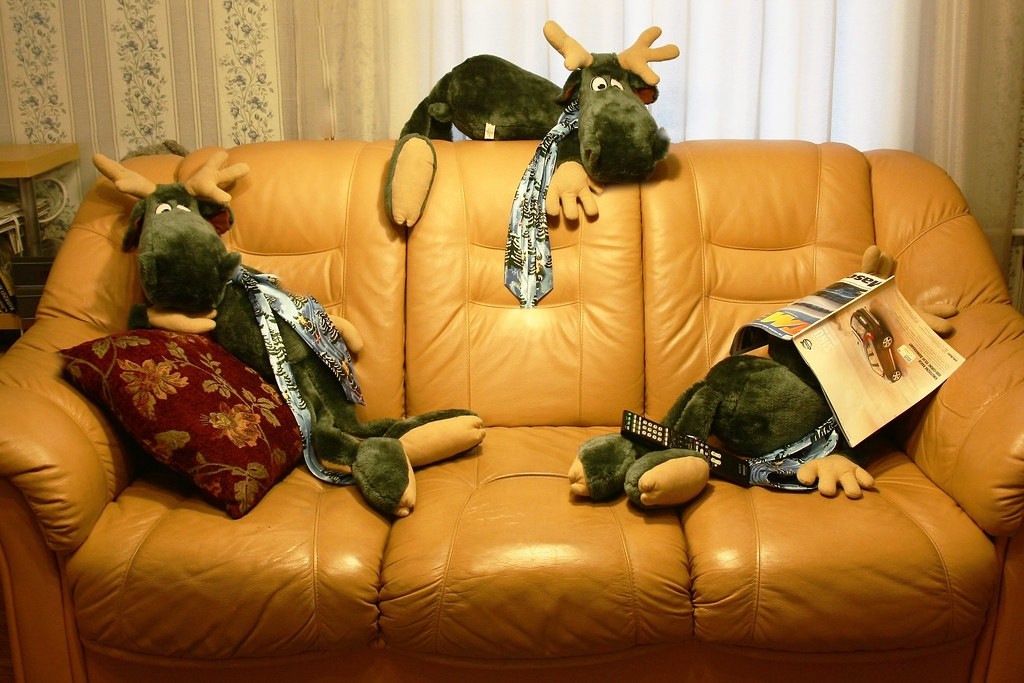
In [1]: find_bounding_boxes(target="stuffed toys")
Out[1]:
[568,246,959,515]
[92,151,486,520]
[383,19,680,219]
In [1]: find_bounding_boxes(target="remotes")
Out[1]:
[620,410,750,488]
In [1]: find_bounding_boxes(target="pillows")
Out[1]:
[54,330,303,520]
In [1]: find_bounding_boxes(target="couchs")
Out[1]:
[0,139,1024,683]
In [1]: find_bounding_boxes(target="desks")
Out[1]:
[0,142,80,258]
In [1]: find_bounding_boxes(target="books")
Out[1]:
[728,272,965,448]
